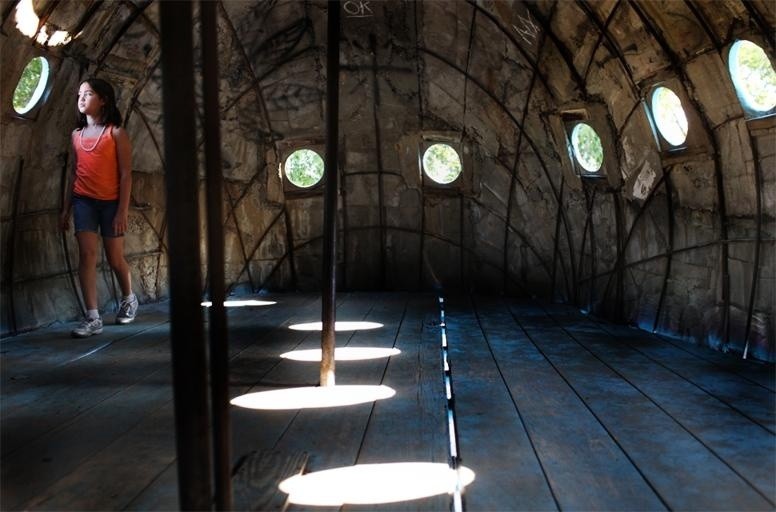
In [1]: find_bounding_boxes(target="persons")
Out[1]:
[59,78,139,338]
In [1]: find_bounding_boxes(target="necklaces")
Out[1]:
[80,124,107,151]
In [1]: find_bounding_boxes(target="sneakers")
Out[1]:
[71,315,103,337]
[116,293,138,324]
